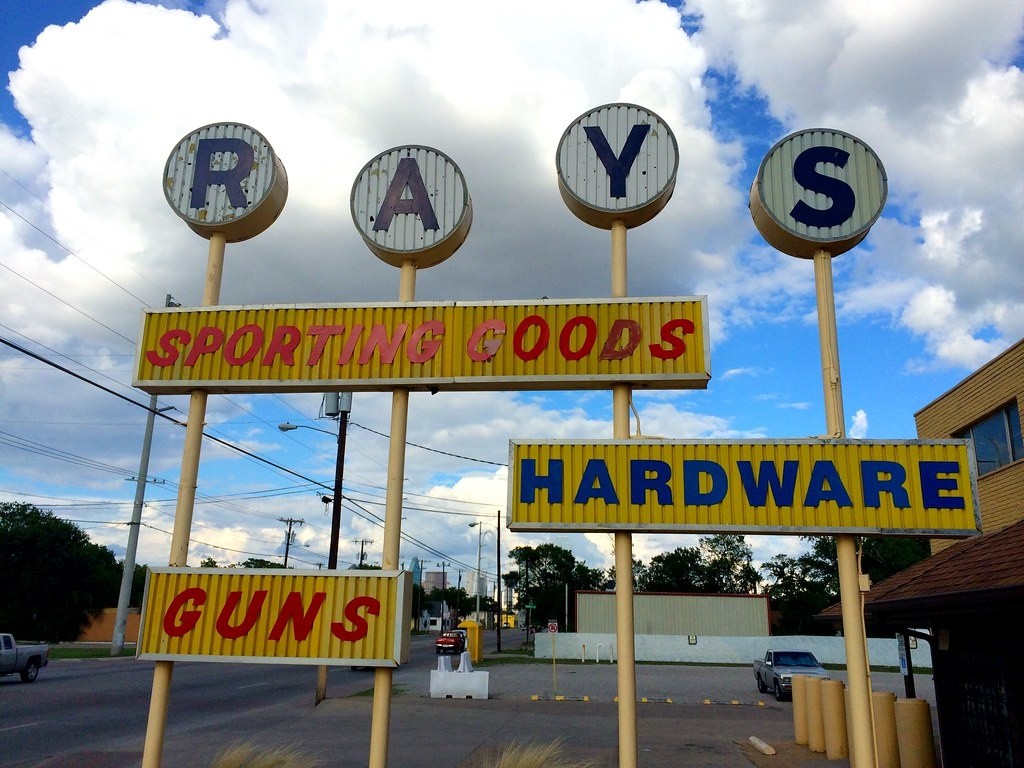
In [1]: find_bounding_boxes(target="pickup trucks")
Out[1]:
[753,648,832,700]
[0,634,52,682]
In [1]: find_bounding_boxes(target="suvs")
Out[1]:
[435,629,467,655]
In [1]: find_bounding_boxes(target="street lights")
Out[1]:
[468,510,501,651]
[278,421,347,569]
